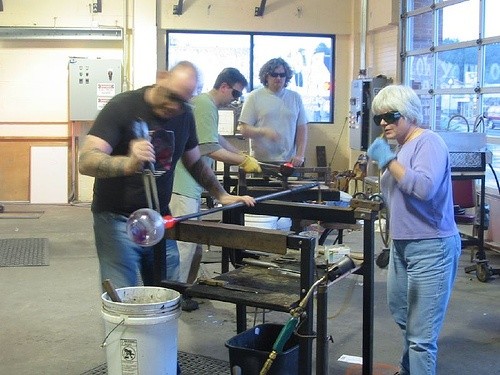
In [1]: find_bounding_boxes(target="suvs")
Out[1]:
[485,106,500,129]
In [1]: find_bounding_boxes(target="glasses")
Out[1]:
[227,82,242,100]
[162,86,188,108]
[269,73,286,77]
[373,110,400,126]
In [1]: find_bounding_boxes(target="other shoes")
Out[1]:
[181,297,199,313]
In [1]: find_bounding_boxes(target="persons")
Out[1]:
[238,58,308,167]
[367,85,462,375]
[78,61,256,375]
[170,67,262,312]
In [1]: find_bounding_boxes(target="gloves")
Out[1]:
[237,150,263,173]
[366,137,397,169]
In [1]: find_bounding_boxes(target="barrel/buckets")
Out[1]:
[101,286,182,375]
